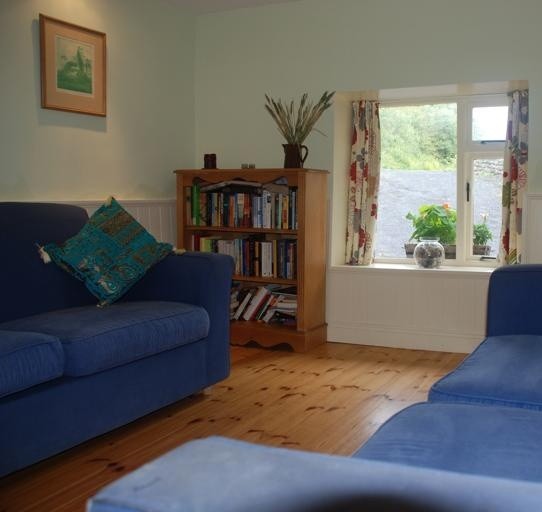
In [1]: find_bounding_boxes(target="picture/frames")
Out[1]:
[39,14,106,119]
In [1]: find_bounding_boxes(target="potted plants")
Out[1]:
[260,91,336,171]
[402,204,490,268]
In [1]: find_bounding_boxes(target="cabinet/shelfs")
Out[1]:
[172,167,334,353]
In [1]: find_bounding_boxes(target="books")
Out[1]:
[188,175,297,330]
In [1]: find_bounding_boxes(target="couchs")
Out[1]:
[0,202,233,477]
[87,263,542,512]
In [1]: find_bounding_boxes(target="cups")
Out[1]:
[249,163,255,168]
[241,163,248,169]
[203,152,217,169]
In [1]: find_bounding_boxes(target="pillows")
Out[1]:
[43,194,174,308]
